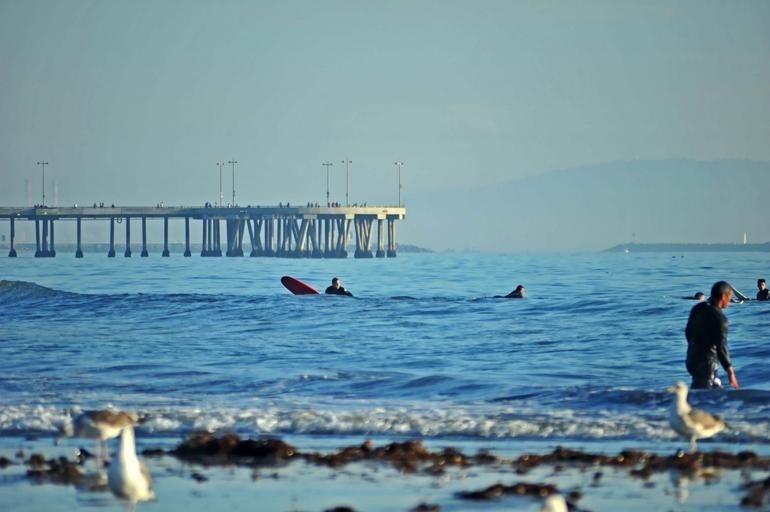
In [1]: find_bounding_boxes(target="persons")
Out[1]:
[694,292,706,301]
[685,281,740,391]
[755,278,770,300]
[506,284,525,298]
[324,278,353,297]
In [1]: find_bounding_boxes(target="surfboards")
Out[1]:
[280,276,319,295]
[731,285,750,302]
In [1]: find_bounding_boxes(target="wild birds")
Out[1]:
[53,409,154,470]
[665,379,735,455]
[107,425,157,512]
